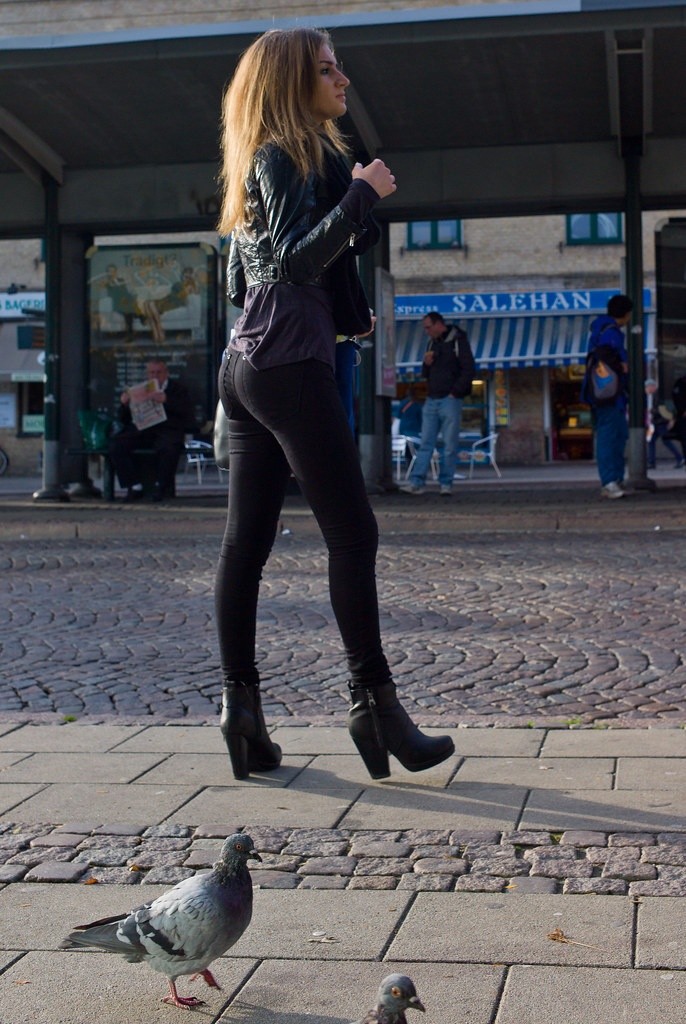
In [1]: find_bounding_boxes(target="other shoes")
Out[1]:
[122,490,144,502]
[674,459,685,468]
[149,485,164,501]
[647,463,656,469]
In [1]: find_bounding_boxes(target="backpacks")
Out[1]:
[583,322,621,406]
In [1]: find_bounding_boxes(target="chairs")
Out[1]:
[405,434,442,480]
[465,431,503,479]
[184,439,223,483]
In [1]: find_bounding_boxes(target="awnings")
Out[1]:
[391,288,658,382]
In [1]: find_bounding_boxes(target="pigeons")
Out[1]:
[350,973,426,1024]
[59,833,263,1010]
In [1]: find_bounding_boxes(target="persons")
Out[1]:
[212,12,455,780]
[110,356,207,502]
[106,263,146,342]
[645,379,685,469]
[143,266,196,342]
[552,367,579,453]
[580,294,636,498]
[392,379,467,480]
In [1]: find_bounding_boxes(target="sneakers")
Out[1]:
[600,482,625,499]
[400,484,426,495]
[440,484,452,494]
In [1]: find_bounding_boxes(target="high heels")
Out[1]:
[347,679,455,780]
[219,683,283,779]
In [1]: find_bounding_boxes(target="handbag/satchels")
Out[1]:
[334,334,363,447]
[664,399,677,416]
